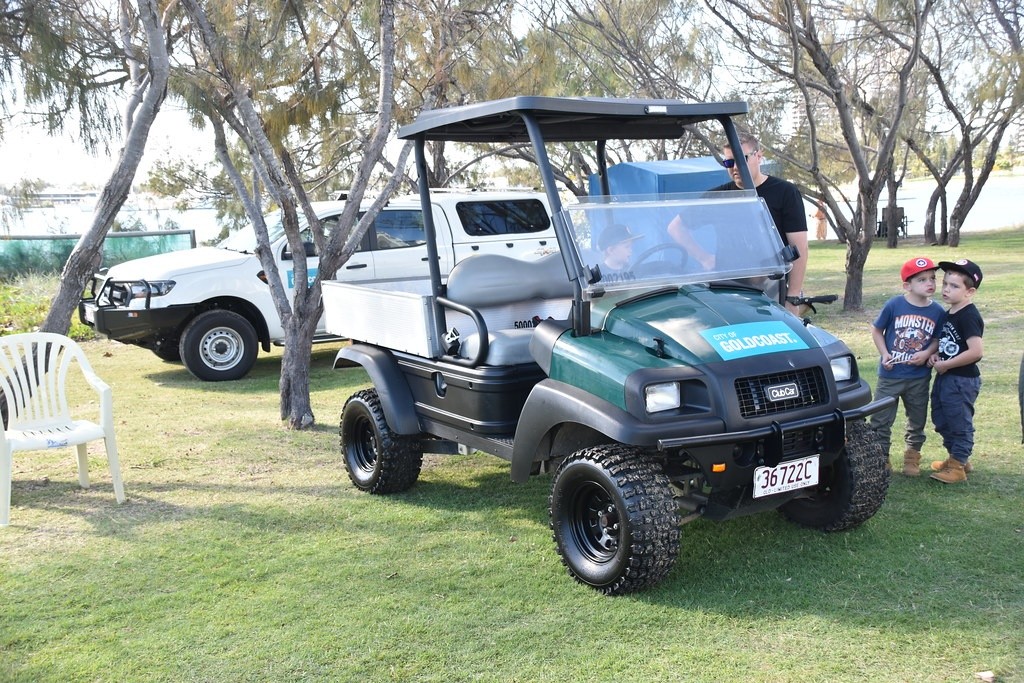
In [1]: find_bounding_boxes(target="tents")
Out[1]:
[589,154,786,270]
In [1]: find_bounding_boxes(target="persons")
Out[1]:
[815,199,827,240]
[870,258,984,482]
[588,224,645,283]
[667,132,809,318]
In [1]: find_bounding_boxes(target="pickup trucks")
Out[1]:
[78,187,578,382]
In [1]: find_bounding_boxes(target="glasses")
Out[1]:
[723,149,757,168]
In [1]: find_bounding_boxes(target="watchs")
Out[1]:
[785,296,801,306]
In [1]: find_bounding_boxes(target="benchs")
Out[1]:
[377,217,424,241]
[445,246,606,368]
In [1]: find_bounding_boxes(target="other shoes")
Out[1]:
[930,459,967,484]
[931,456,973,473]
[903,448,921,477]
[883,458,891,471]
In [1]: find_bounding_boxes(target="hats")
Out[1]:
[939,259,983,289]
[901,257,940,282]
[597,224,644,251]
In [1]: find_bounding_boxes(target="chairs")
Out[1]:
[851,207,913,239]
[304,242,318,256]
[0,333,125,525]
